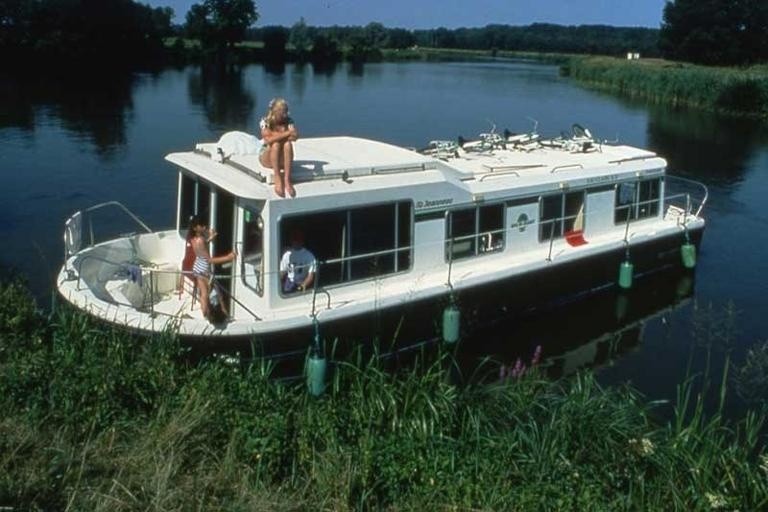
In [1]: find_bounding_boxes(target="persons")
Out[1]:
[281,231,317,293]
[260,95,300,199]
[187,214,235,317]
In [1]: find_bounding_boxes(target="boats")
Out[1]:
[385,266,699,396]
[55,118,709,381]
